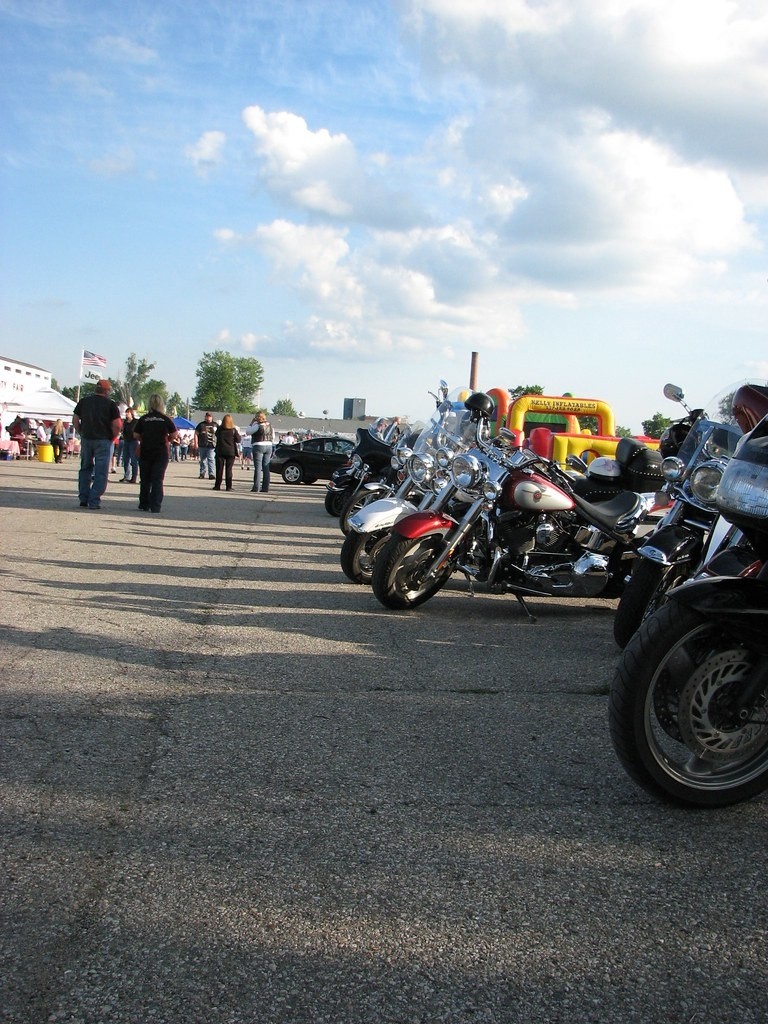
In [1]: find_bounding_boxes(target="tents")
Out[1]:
[7,386,82,457]
[118,402,201,430]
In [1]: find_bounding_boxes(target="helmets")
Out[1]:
[659,424,697,460]
[588,457,621,482]
[465,393,494,418]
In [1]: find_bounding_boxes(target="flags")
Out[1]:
[82,350,106,367]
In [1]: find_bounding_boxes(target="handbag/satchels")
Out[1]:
[235,442,239,457]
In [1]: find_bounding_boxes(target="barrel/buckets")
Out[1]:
[37,445,54,462]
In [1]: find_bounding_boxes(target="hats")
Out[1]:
[96,380,110,389]
[36,420,44,425]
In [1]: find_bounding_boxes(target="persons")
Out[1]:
[5,412,316,491]
[72,380,123,509]
[133,393,178,513]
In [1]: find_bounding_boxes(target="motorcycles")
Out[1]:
[324,377,767,807]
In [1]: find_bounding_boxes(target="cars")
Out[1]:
[266,436,356,486]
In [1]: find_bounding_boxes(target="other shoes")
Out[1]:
[241,465,244,469]
[226,489,234,492]
[213,487,220,490]
[111,467,116,473]
[80,502,88,506]
[120,478,129,482]
[199,475,204,479]
[129,479,136,483]
[209,475,215,478]
[247,466,250,470]
